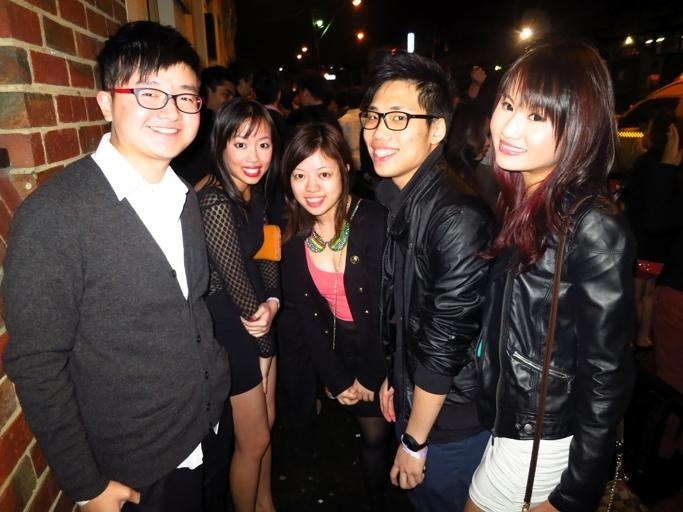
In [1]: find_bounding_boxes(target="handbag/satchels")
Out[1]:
[596,477,644,512]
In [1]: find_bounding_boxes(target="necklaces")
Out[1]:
[307,221,350,253]
[314,196,361,348]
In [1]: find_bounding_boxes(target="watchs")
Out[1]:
[399,433,428,451]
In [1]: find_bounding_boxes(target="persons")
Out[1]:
[193,97,283,511]
[627,111,681,347]
[283,72,362,169]
[0,21,233,511]
[447,65,492,179]
[360,54,496,511]
[466,40,637,511]
[637,122,681,481]
[274,122,387,512]
[180,65,282,167]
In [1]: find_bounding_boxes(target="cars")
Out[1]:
[612,79,683,178]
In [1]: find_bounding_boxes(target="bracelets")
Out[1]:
[265,297,279,309]
[402,441,427,458]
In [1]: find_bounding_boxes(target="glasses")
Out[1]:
[358,109,437,131]
[112,88,204,114]
